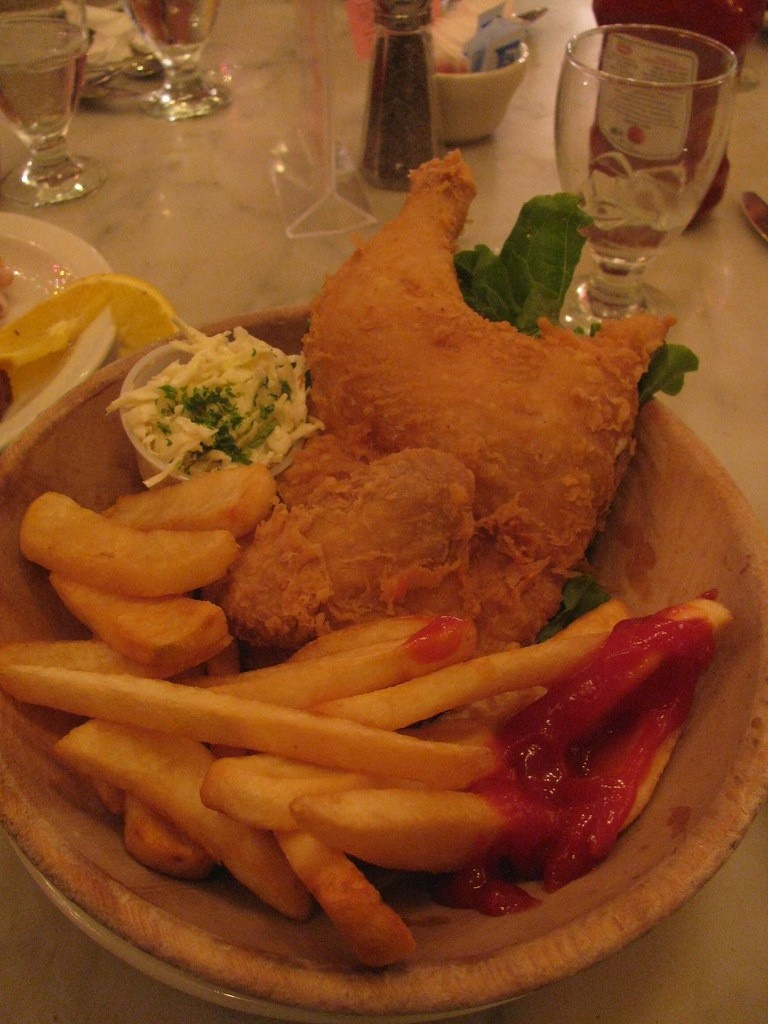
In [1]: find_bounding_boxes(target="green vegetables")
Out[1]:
[155,349,293,466]
[454,189,702,643]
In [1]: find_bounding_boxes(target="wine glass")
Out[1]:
[0,0,108,208]
[119,0,234,121]
[552,24,739,336]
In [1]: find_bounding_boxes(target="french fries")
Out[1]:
[0,463,732,969]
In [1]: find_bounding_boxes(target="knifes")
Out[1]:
[738,190,768,244]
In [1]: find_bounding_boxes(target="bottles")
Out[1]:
[361,0,434,191]
[590,0,768,224]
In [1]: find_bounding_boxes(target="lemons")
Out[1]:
[0,273,185,420]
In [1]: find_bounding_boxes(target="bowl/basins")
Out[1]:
[0,211,115,445]
[0,303,768,1024]
[433,41,529,148]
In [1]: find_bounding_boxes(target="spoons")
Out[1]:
[73,54,165,104]
